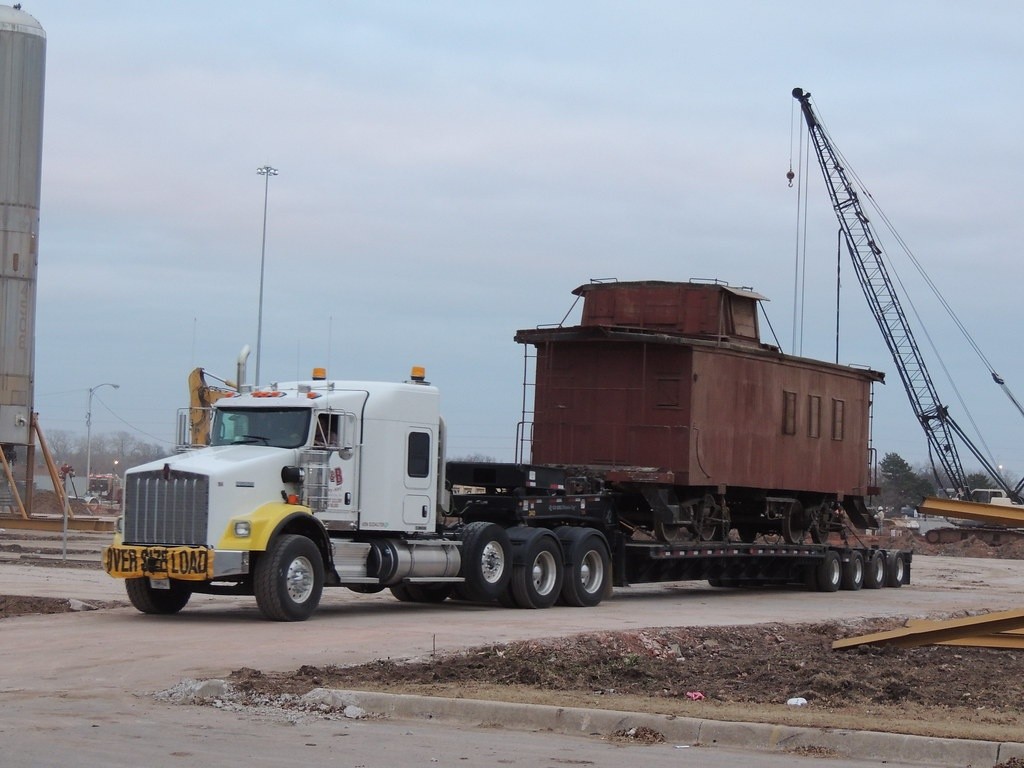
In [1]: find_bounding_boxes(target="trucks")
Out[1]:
[85,473,124,514]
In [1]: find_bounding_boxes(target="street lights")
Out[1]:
[254,167,279,390]
[86,382,119,494]
[111,459,120,504]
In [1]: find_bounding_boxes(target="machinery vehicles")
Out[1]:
[784,86,1024,547]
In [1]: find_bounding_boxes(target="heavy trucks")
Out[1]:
[102,345,916,623]
